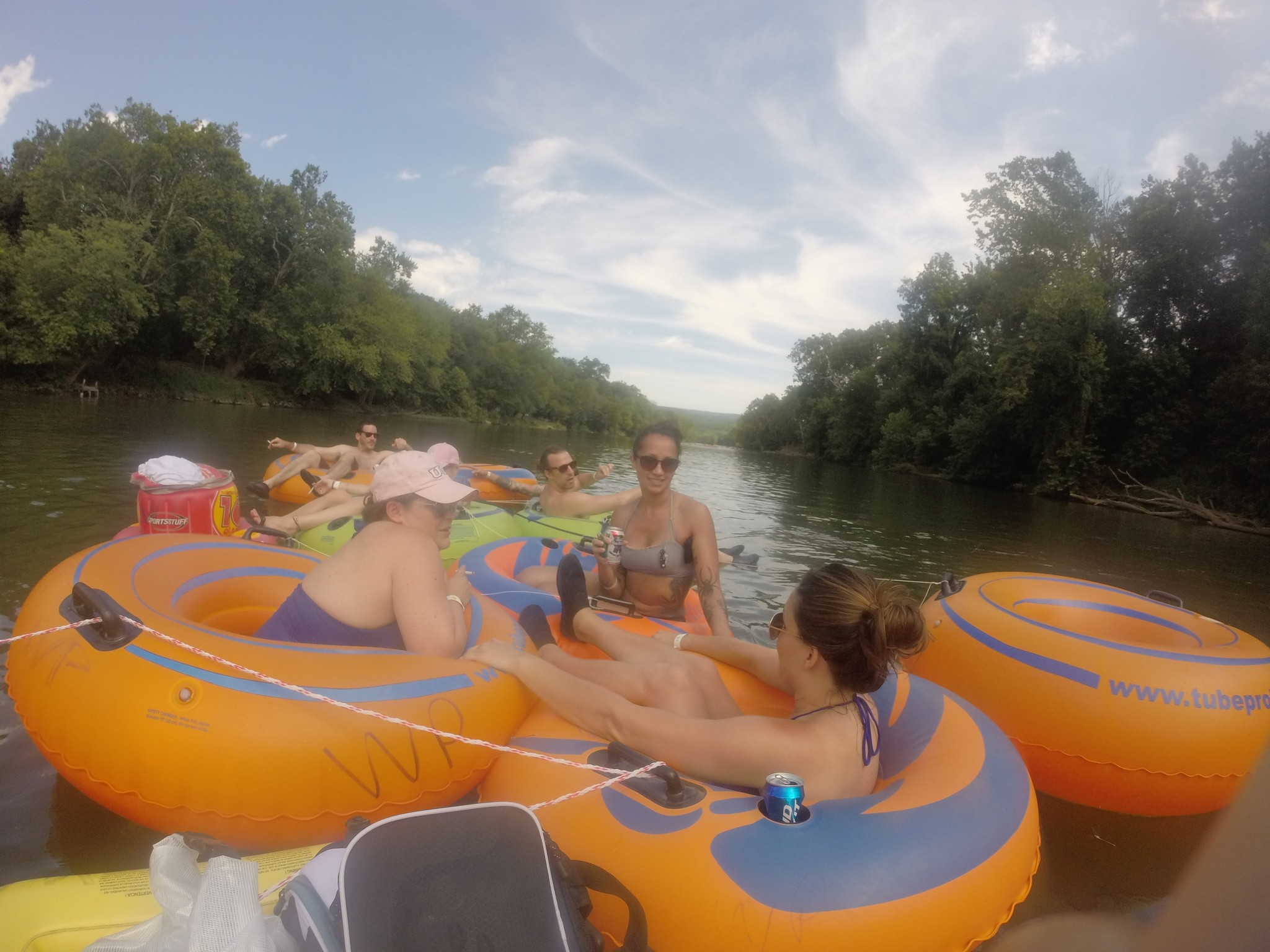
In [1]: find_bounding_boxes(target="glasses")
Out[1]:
[768,612,803,640]
[633,453,681,473]
[407,501,457,518]
[546,460,577,473]
[360,431,379,439]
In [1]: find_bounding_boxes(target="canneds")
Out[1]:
[601,527,625,563]
[762,773,805,823]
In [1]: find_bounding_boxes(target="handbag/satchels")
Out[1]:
[272,802,604,952]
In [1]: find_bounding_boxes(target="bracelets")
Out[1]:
[291,443,297,451]
[486,472,491,479]
[332,480,335,488]
[598,575,618,590]
[334,481,340,490]
[674,634,687,650]
[594,473,598,481]
[447,595,465,611]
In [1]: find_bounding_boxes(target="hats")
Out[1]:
[427,442,460,467]
[369,450,480,505]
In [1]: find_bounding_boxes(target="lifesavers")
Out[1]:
[454,461,540,501]
[8,533,538,847]
[508,505,615,547]
[108,510,280,547]
[260,452,378,505]
[1,840,370,951]
[279,500,521,573]
[445,537,733,651]
[895,572,1269,814]
[480,644,1042,952]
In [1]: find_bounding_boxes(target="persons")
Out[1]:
[252,451,472,659]
[515,423,731,638]
[537,445,642,519]
[458,553,928,806]
[246,421,578,539]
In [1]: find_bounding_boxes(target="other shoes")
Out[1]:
[248,482,269,499]
[556,553,590,644]
[719,544,745,556]
[732,554,759,565]
[300,469,324,497]
[518,604,557,651]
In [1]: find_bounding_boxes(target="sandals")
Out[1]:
[244,508,303,537]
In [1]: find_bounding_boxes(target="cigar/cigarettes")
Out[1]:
[309,486,314,494]
[268,440,274,446]
[455,569,476,575]
